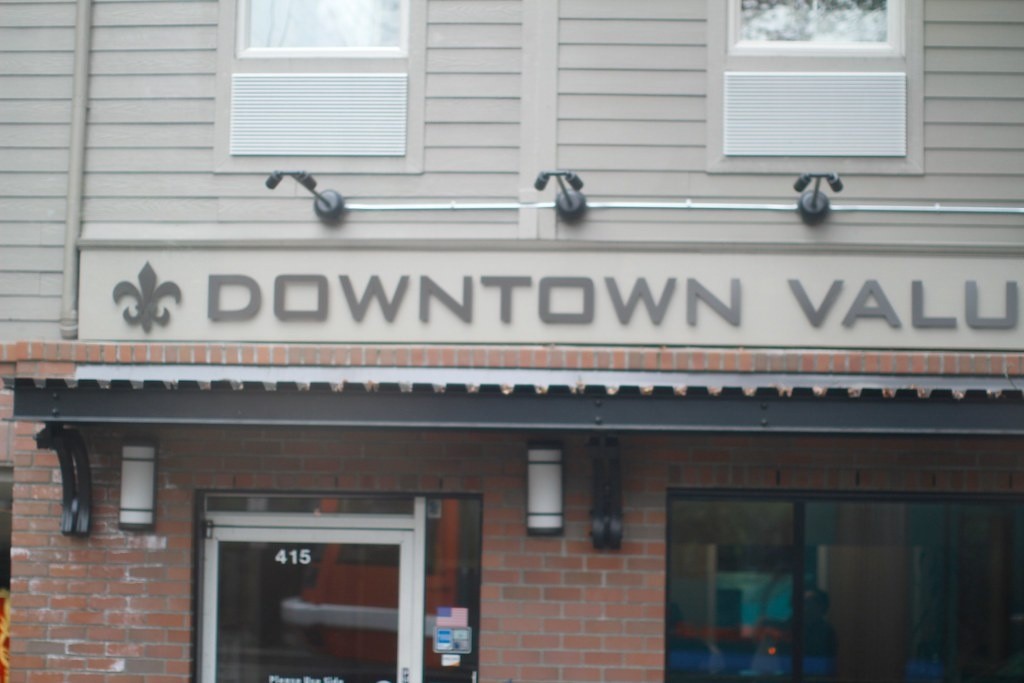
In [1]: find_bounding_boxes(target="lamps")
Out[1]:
[791,173,845,223]
[266,168,343,219]
[120,438,159,530]
[528,440,565,535]
[532,172,590,220]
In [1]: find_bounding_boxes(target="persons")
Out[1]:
[779,585,837,676]
